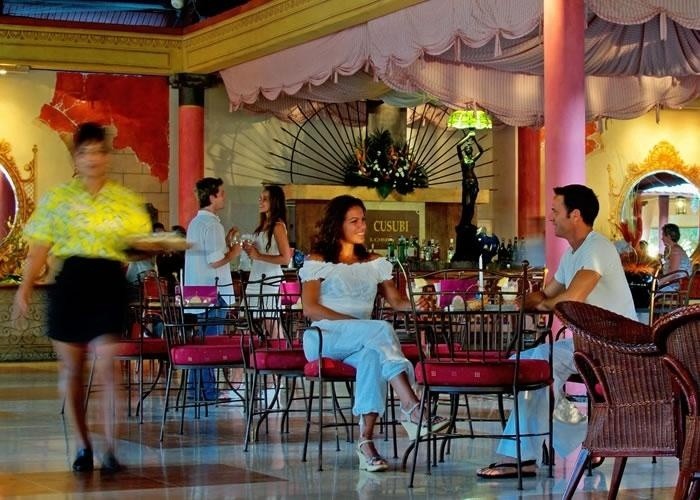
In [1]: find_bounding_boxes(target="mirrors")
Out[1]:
[609,139,699,263]
[1,137,39,285]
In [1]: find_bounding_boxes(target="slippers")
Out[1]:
[476,459,538,480]
[204,391,231,401]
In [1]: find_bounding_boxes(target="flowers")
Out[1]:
[347,126,427,197]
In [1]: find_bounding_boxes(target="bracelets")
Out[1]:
[540,291,548,299]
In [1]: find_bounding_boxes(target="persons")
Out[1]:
[10,122,177,471]
[155,226,186,336]
[220,185,291,398]
[475,184,640,480]
[648,224,694,310]
[183,177,236,401]
[125,223,167,285]
[298,195,450,473]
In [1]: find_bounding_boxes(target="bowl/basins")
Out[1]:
[465,300,481,312]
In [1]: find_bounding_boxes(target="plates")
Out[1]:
[125,237,194,253]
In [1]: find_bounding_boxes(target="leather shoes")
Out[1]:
[101,461,127,477]
[72,455,95,473]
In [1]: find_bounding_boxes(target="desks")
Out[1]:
[382,299,526,475]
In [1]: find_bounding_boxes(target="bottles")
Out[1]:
[498,235,528,270]
[447,237,456,264]
[388,234,442,263]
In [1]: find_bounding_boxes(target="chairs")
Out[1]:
[615,252,700,317]
[412,266,479,301]
[82,252,406,473]
[551,295,700,499]
[486,261,530,302]
[399,260,530,493]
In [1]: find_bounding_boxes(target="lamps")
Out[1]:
[675,196,688,216]
[446,99,494,130]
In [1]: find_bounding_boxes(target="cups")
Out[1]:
[502,282,518,303]
[230,234,256,248]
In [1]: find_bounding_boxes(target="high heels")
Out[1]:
[356,438,389,473]
[399,401,450,442]
